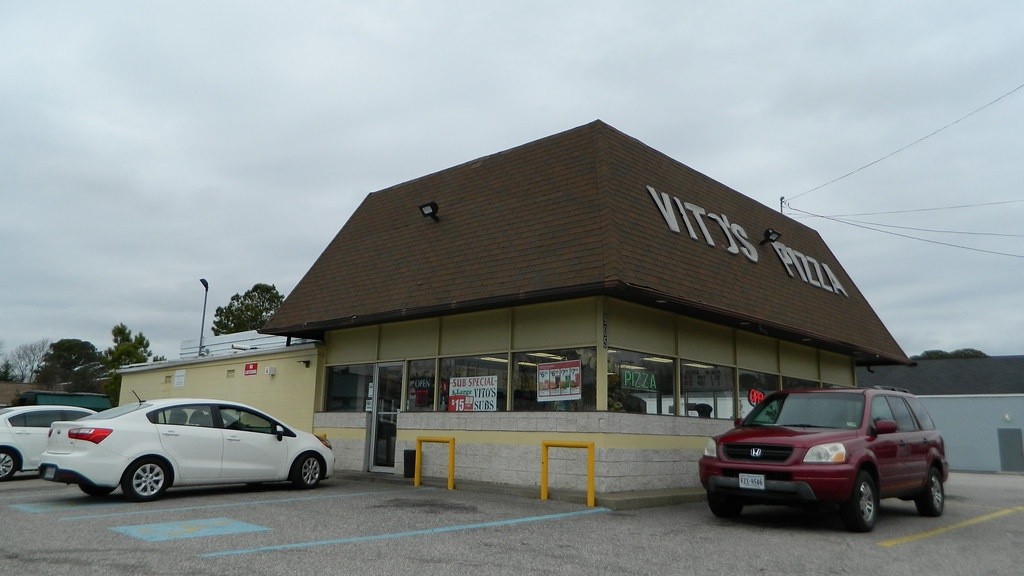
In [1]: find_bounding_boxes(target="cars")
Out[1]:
[0,404,101,481]
[39,397,337,503]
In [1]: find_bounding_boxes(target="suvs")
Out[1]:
[697,383,951,532]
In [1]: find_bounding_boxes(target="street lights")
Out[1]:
[198,278,209,356]
